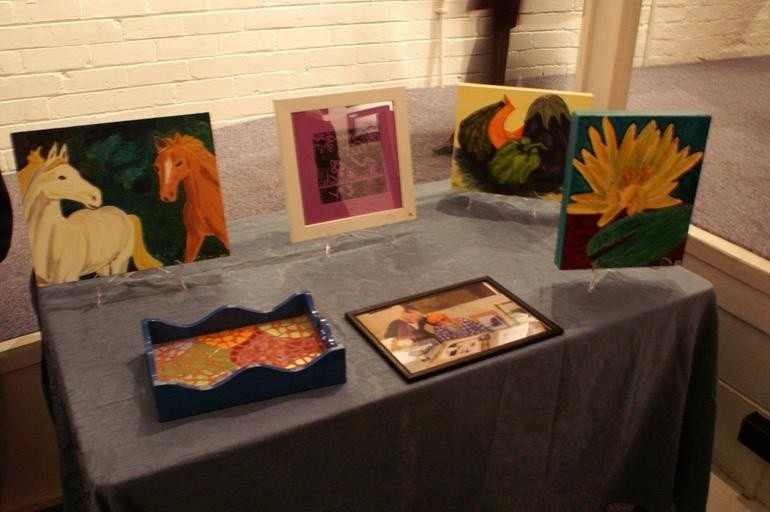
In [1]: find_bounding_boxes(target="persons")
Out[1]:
[381,305,441,349]
[425,311,495,344]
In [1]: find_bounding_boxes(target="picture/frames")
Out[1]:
[344,273,565,385]
[270,86,418,245]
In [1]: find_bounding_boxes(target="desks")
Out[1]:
[29,188,718,512]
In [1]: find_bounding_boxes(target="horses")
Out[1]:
[16,141,164,288]
[151,131,230,262]
